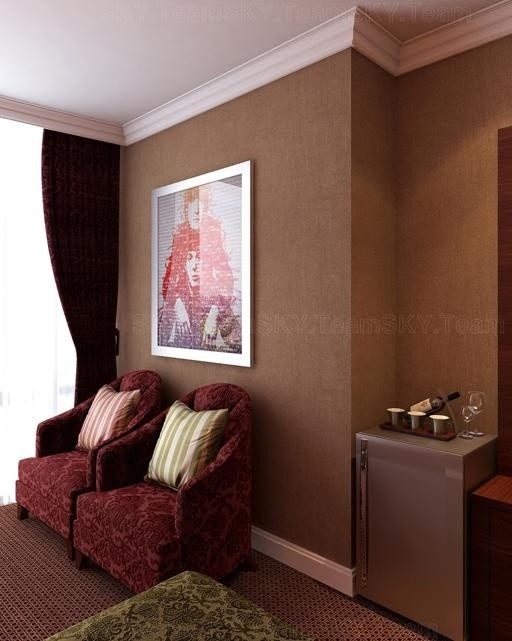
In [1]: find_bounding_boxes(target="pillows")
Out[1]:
[143,399,230,491]
[75,383,143,452]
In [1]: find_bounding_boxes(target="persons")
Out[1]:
[157,240,241,353]
[162,187,234,350]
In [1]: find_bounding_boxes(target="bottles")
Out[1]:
[402,391,462,426]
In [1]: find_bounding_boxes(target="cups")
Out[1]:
[386,407,448,435]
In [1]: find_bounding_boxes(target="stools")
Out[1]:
[43,571,317,641]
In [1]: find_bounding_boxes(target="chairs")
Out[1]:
[15,369,163,560]
[72,383,256,594]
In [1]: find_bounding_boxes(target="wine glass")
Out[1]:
[460,390,487,438]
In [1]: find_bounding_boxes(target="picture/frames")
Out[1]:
[151,160,256,368]
